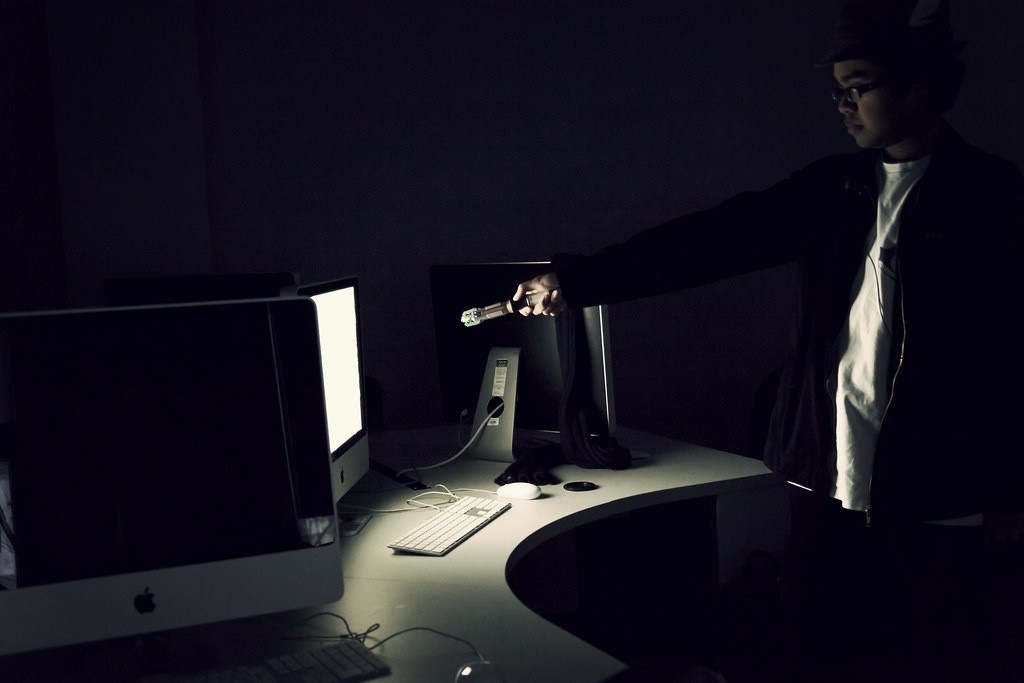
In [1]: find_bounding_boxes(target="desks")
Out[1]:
[0,415,774,683]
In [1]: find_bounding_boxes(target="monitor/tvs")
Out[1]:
[296,276,373,537]
[428,257,616,462]
[0,296,347,683]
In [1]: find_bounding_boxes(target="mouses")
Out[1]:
[496,482,541,499]
[454,660,505,683]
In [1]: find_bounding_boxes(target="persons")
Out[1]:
[512,0,1024,683]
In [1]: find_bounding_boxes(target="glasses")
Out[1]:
[830,74,893,106]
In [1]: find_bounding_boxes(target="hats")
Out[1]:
[815,0,970,64]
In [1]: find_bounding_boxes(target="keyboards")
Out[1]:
[387,495,511,556]
[187,639,391,683]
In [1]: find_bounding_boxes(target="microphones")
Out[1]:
[461,290,545,327]
